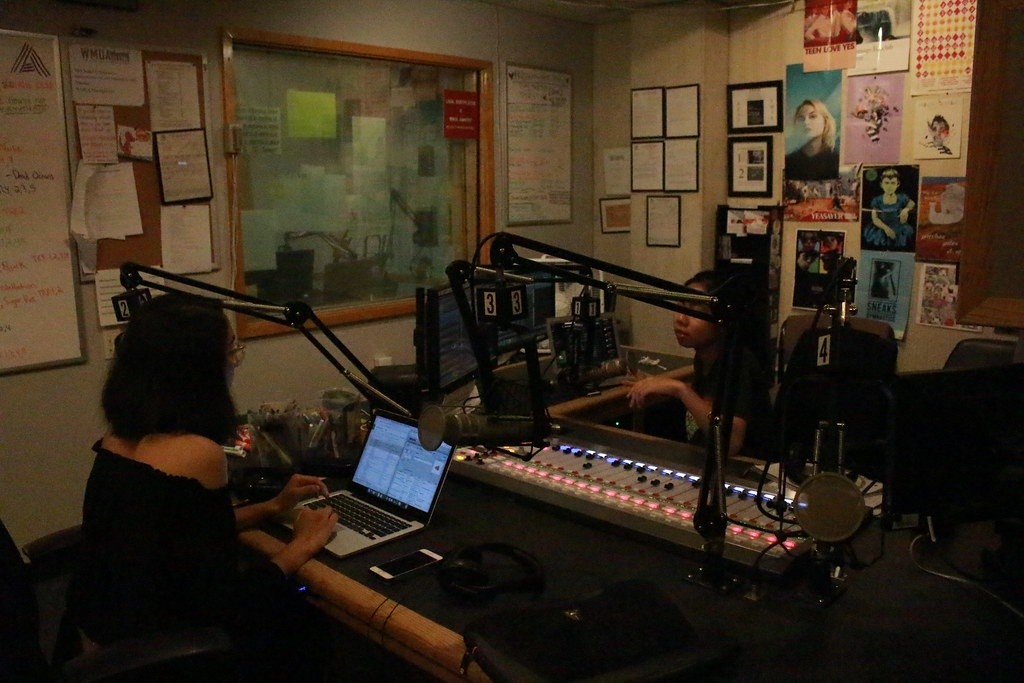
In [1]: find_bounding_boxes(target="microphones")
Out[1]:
[794,474,866,542]
[547,359,627,388]
[417,407,563,453]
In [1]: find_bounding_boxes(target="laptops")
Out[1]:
[279,408,457,558]
[547,312,636,387]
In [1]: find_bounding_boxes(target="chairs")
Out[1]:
[0,518,247,683]
[759,318,1017,463]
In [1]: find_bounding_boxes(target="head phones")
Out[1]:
[439,541,547,604]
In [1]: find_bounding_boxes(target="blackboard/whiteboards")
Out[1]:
[0,25,91,377]
[505,60,574,226]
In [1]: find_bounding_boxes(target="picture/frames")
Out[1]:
[600,196,631,235]
[726,80,784,133]
[727,135,774,198]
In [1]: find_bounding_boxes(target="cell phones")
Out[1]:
[369,548,444,582]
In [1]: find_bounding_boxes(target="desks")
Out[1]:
[237,346,1024,683]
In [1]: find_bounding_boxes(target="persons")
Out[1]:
[622,269,780,461]
[804,0,857,40]
[81,292,401,683]
[796,231,843,273]
[785,98,840,180]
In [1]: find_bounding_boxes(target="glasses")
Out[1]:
[223,342,246,369]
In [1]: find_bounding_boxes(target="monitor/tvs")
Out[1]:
[477,269,555,354]
[879,362,1024,530]
[425,276,497,397]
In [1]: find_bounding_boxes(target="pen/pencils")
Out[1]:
[247,400,330,465]
